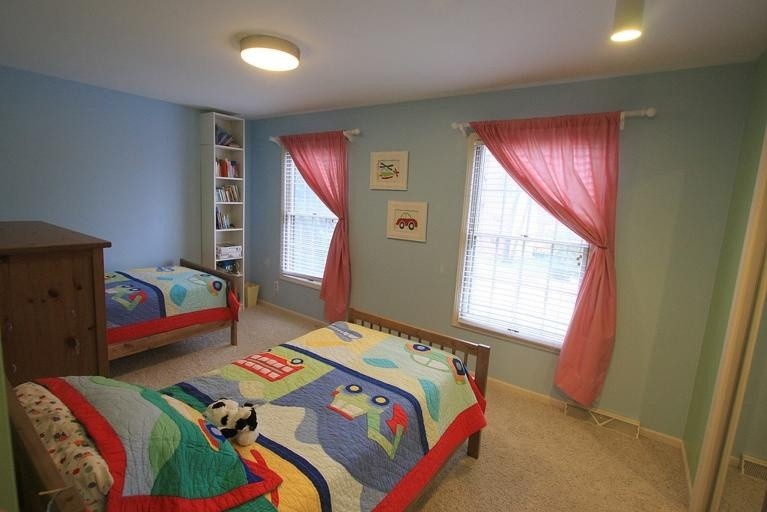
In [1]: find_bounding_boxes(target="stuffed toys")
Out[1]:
[204,398,260,446]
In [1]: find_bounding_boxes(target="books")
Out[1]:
[215,125,242,229]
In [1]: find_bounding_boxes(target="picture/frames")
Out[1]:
[367,150,429,244]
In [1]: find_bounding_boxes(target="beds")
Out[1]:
[104,255,241,362]
[0,306,492,512]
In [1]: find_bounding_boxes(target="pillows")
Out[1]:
[32,370,282,511]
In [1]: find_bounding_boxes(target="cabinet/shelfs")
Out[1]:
[197,110,246,313]
[1,219,113,386]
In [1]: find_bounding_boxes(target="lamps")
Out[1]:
[608,0,646,44]
[238,33,300,73]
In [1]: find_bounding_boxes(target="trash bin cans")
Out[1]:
[245,280,260,308]
